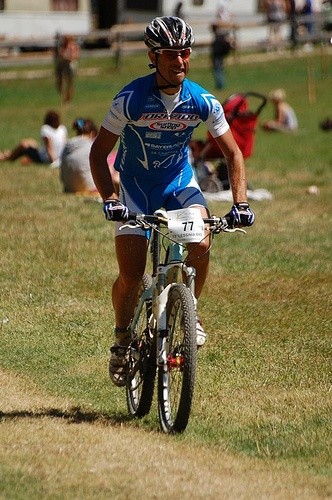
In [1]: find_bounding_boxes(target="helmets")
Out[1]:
[144,16,196,87]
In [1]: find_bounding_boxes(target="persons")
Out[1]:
[0,3,304,200]
[88,15,256,387]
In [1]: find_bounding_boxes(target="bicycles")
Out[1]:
[109,211,248,437]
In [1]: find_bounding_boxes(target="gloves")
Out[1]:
[225,202,256,227]
[102,200,131,223]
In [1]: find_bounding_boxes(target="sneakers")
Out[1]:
[107,342,133,388]
[180,314,207,346]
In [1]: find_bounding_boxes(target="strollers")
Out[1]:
[188,91,268,193]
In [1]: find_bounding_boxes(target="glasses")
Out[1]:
[150,48,193,60]
